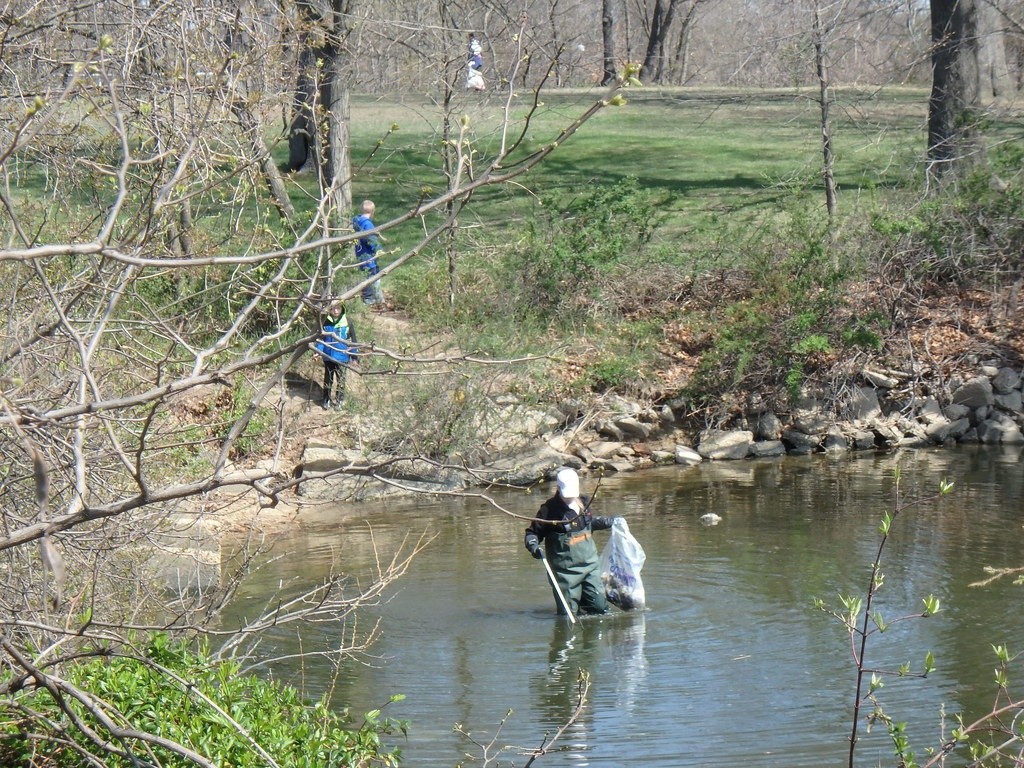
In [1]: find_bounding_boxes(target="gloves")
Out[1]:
[594,515,623,529]
[525,536,543,560]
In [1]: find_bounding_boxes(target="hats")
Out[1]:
[556,469,579,499]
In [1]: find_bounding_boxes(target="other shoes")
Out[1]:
[322,399,330,409]
[334,400,342,411]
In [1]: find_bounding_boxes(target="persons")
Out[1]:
[524,469,623,615]
[313,301,359,409]
[466,33,485,90]
[352,199,394,312]
